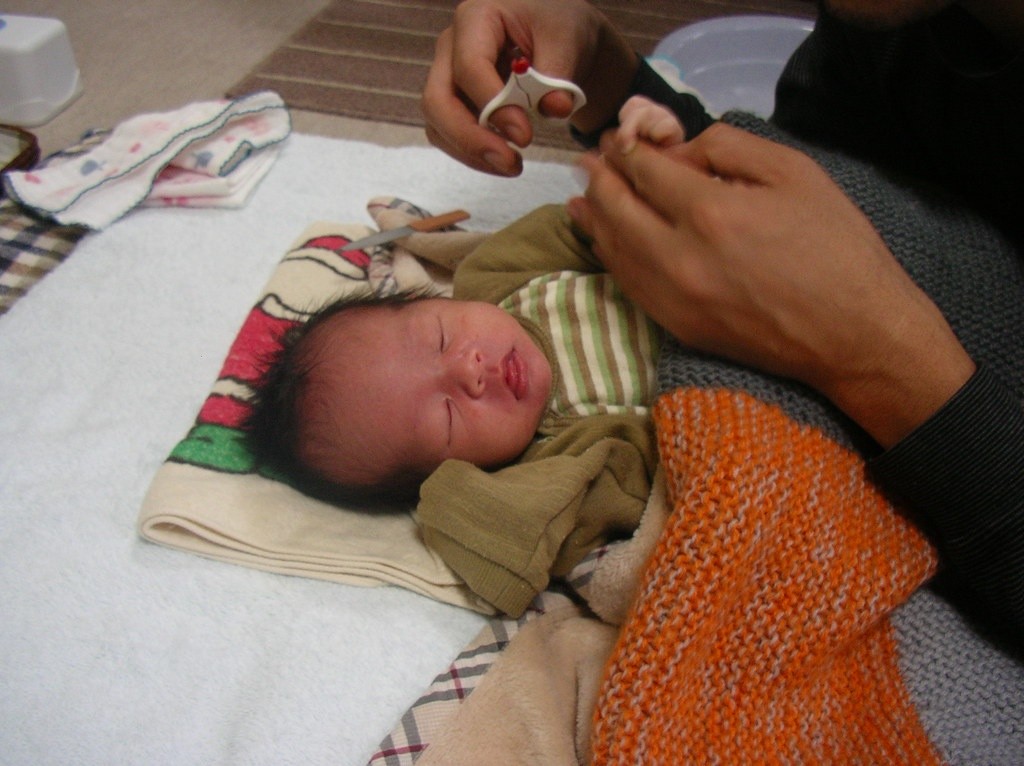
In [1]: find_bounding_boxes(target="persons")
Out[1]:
[420,0,1024,664]
[234,96,688,621]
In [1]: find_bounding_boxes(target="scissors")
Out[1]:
[478,46,588,141]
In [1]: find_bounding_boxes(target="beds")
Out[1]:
[0,132,1024,766]
